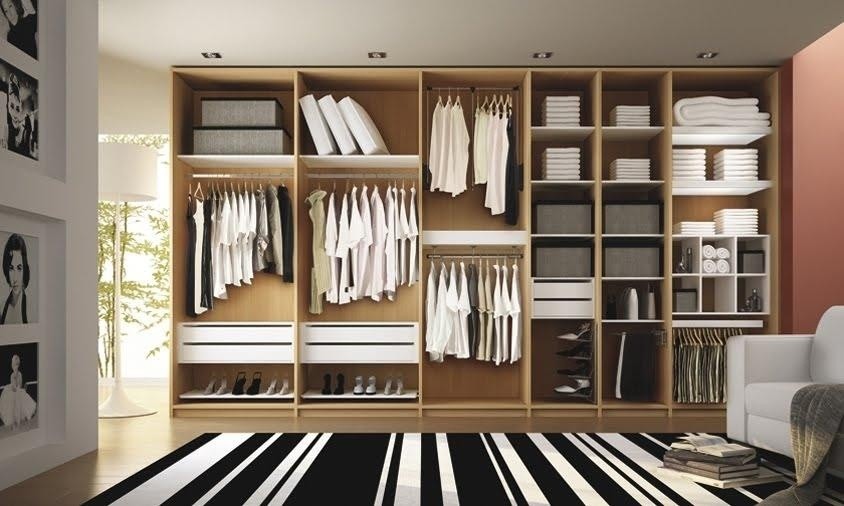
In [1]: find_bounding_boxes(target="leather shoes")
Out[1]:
[353,376,376,394]
[321,374,344,394]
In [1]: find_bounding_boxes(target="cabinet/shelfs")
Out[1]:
[169,67,782,432]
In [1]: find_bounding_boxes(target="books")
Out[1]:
[657,428,784,489]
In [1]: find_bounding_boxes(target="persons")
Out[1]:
[3,73,35,157]
[0,233,34,325]
[0,354,37,430]
[1,0,39,60]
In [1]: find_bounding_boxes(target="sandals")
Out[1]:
[217,376,227,394]
[280,378,289,394]
[204,376,216,394]
[384,376,403,395]
[232,372,246,395]
[247,372,261,394]
[267,378,277,394]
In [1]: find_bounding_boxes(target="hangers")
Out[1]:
[670,323,743,346]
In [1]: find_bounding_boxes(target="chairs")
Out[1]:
[725,306,844,481]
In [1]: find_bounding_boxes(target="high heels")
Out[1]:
[554,323,590,394]
